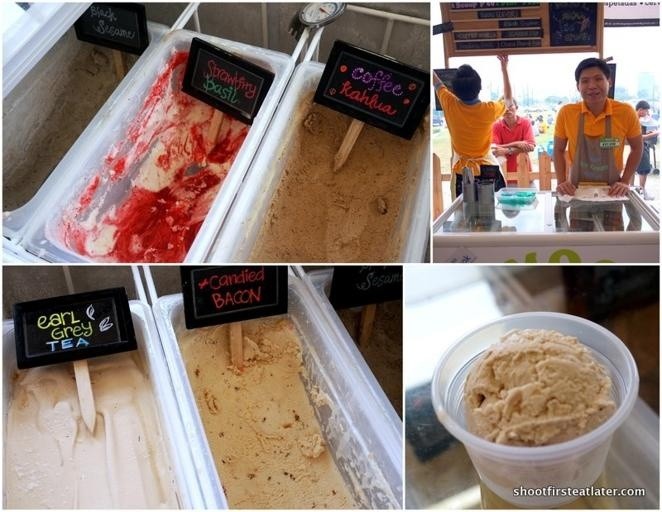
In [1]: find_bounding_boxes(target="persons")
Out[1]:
[554,198,642,233]
[553,57,645,197]
[521,100,659,161]
[629,100,659,199]
[430,53,515,198]
[489,96,538,188]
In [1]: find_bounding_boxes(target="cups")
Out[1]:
[430,309,641,508]
[462,179,495,220]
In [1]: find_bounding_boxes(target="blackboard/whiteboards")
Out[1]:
[180,264,288,329]
[313,39,430,141]
[548,1,599,53]
[75,1,149,57]
[181,37,275,125]
[329,266,402,309]
[14,286,138,369]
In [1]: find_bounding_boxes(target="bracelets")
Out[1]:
[507,147,512,155]
